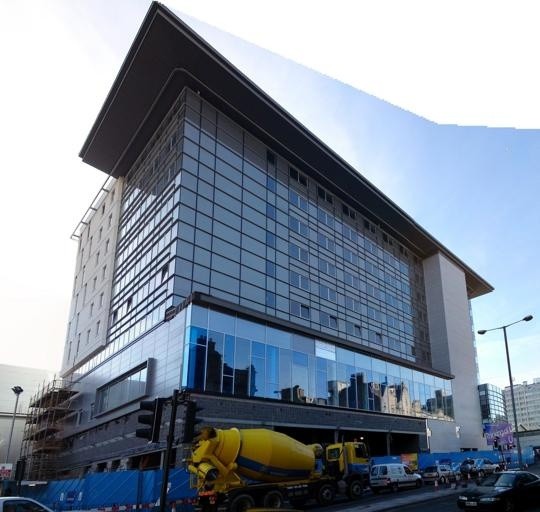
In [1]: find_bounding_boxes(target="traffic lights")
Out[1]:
[185,399,206,441]
[137,395,161,443]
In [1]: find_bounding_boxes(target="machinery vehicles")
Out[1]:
[180,426,374,511]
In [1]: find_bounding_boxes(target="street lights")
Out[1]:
[476,314,536,469]
[2,385,23,464]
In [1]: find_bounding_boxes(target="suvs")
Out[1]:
[461,457,500,481]
[421,465,461,487]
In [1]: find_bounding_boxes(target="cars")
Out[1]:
[460,470,540,511]
[0,497,56,512]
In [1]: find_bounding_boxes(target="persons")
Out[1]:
[441,471,461,489]
[434,478,439,491]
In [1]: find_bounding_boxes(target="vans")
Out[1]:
[369,463,422,492]
